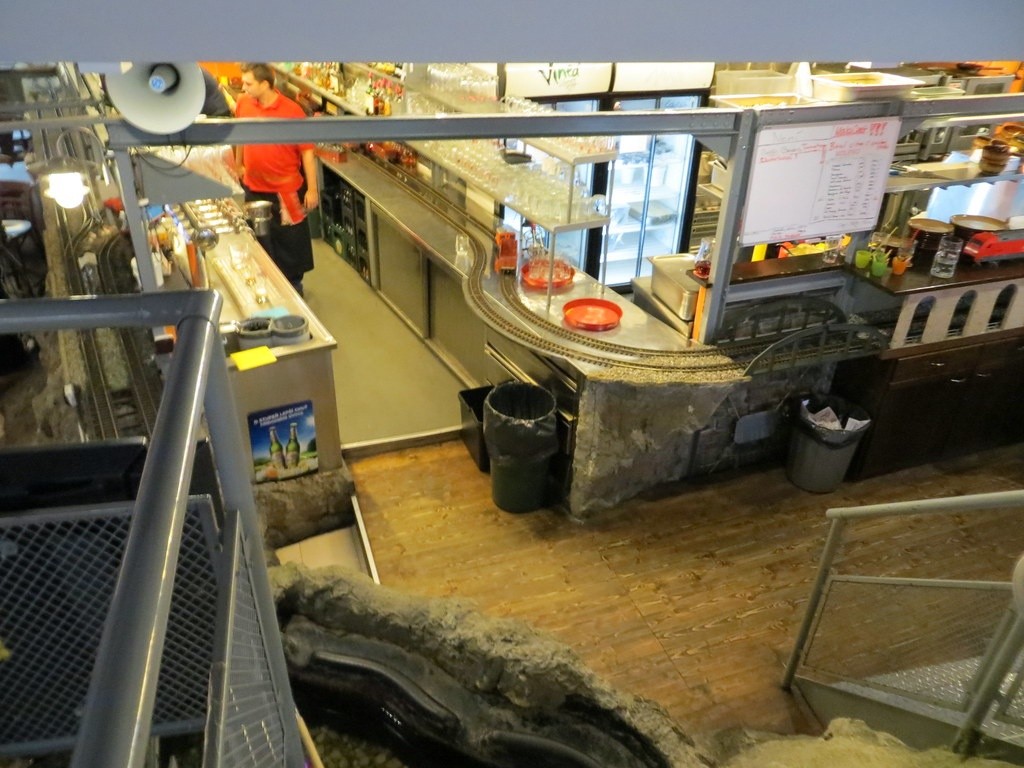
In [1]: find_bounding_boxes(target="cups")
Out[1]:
[822,234,843,263]
[871,253,889,277]
[407,94,436,115]
[855,250,871,269]
[425,136,616,225]
[892,257,910,275]
[229,244,268,304]
[930,235,964,279]
[529,248,571,281]
[694,237,717,276]
[897,238,919,262]
[426,63,553,114]
[456,234,470,255]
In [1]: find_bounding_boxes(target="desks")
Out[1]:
[156,196,343,483]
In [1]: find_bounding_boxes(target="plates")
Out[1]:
[908,218,954,254]
[950,214,1010,237]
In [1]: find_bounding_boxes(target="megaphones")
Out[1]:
[105,62,207,136]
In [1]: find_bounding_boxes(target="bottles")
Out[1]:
[364,72,401,115]
[282,62,344,96]
[325,185,369,279]
[269,427,285,470]
[286,422,300,469]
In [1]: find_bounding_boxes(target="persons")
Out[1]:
[0,285,40,375]
[201,65,230,120]
[232,63,320,298]
[0,71,27,197]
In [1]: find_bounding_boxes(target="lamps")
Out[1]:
[36,127,116,210]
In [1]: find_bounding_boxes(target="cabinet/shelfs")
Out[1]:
[269,61,619,307]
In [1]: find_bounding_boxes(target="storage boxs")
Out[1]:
[810,72,926,102]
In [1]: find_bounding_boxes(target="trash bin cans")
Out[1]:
[788,392,871,497]
[457,383,489,475]
[484,381,561,512]
[235,316,309,352]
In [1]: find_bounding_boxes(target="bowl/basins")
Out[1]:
[979,158,1007,176]
[971,124,1024,155]
[981,145,1009,161]
